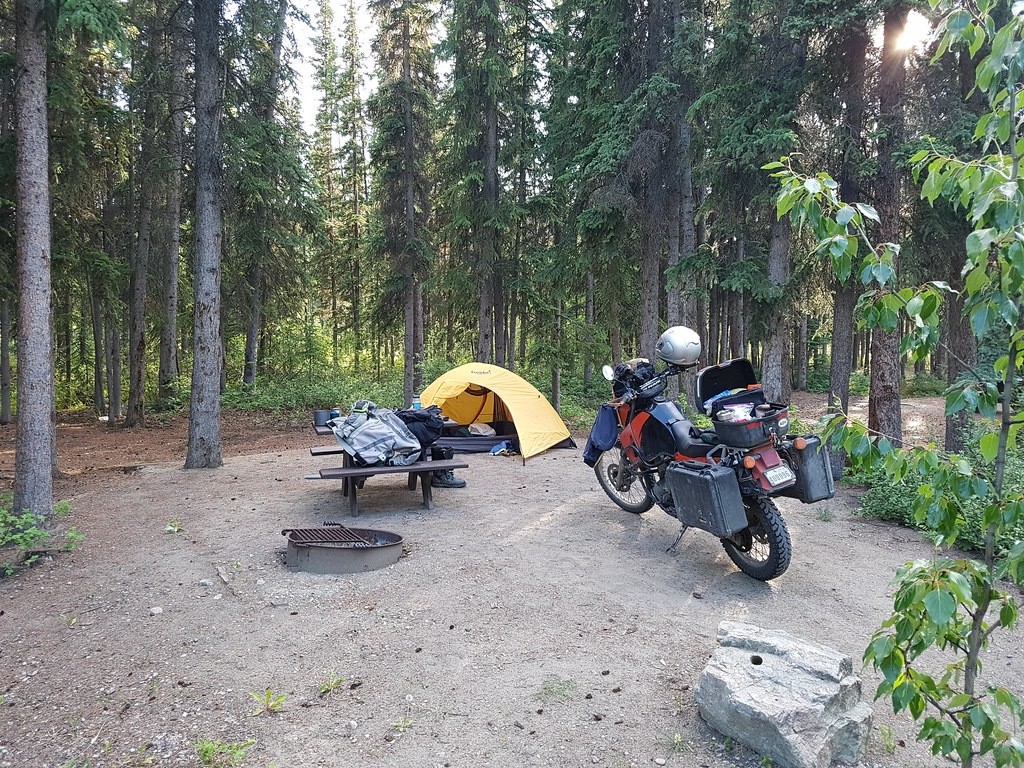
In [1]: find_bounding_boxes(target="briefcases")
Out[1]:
[667,460,748,539]
[773,433,834,504]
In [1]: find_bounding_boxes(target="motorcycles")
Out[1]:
[583,357,837,582]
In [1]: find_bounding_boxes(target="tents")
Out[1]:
[410,362,571,459]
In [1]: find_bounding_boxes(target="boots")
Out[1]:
[431,446,466,488]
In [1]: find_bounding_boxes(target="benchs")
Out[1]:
[319,458,468,480]
[310,441,438,456]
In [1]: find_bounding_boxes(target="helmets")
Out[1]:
[655,326,702,365]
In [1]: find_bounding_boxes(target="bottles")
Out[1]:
[334,405,342,417]
[412,395,421,409]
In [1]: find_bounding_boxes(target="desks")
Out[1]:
[312,414,458,518]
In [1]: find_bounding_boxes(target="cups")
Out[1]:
[314,410,330,425]
[333,408,340,416]
[716,409,737,422]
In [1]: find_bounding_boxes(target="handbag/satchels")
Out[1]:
[394,404,445,453]
[467,423,496,436]
